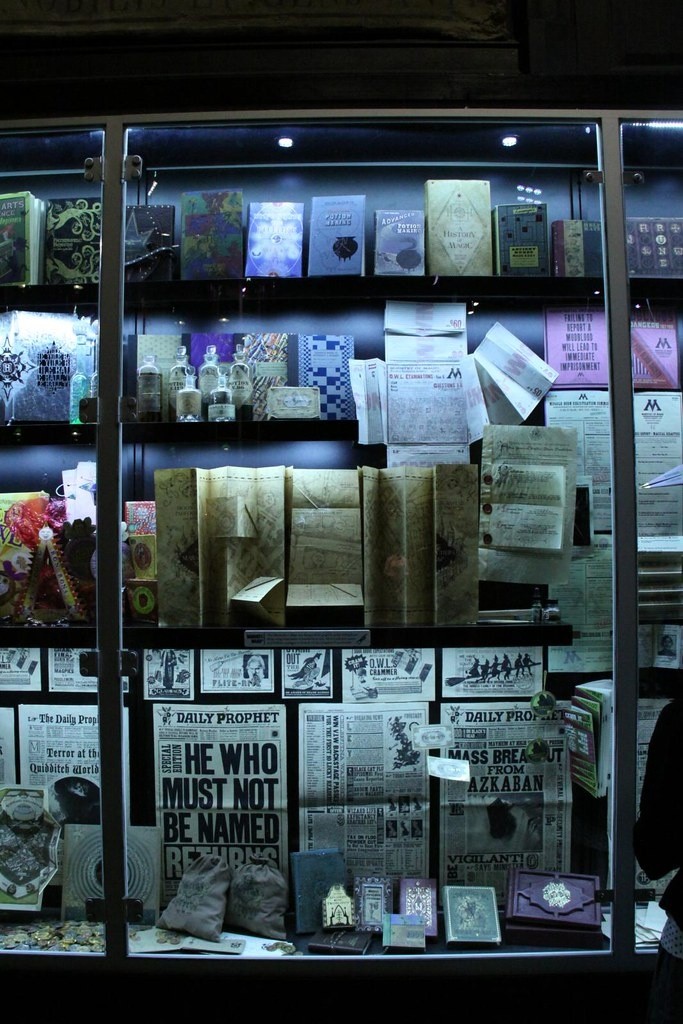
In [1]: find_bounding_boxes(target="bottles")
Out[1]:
[230,344,253,421]
[138,355,160,421]
[531,588,542,624]
[69,334,90,424]
[544,599,561,624]
[208,366,235,421]
[199,345,220,420]
[176,366,201,422]
[168,346,191,422]
[90,372,98,398]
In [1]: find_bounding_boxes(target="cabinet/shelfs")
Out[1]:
[0,110,683,976]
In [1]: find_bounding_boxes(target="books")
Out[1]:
[0,182,682,283]
[564,686,612,798]
[287,846,504,954]
